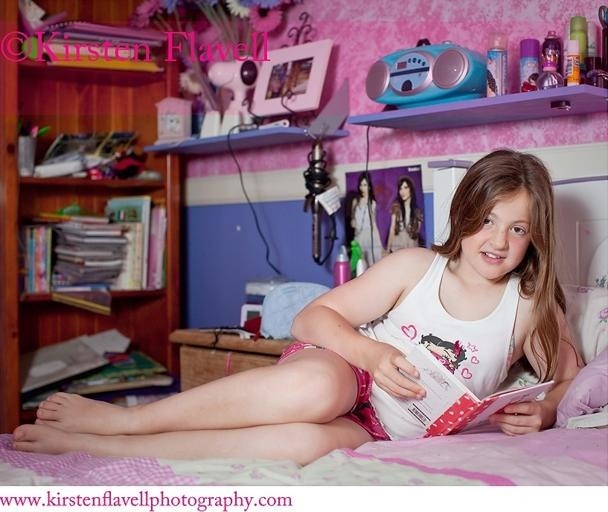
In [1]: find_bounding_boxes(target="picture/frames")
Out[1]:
[249,37,335,119]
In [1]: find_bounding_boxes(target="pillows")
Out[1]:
[554,275,606,427]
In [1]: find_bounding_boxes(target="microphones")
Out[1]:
[311,144,325,262]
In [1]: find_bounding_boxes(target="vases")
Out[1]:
[198,99,246,138]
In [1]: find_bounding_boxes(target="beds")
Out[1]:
[1,349,607,484]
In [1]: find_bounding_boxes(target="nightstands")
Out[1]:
[163,319,306,408]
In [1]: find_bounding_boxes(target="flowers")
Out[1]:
[134,0,290,113]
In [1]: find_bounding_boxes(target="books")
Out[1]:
[17,128,174,412]
[367,342,556,442]
[38,21,168,63]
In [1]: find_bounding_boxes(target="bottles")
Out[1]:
[486,16,588,98]
[334,246,351,288]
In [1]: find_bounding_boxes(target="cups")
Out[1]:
[19,136,36,177]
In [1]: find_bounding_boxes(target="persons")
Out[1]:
[348,171,383,267]
[11,149,586,464]
[385,177,424,253]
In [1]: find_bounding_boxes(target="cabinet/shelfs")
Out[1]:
[7,0,182,416]
